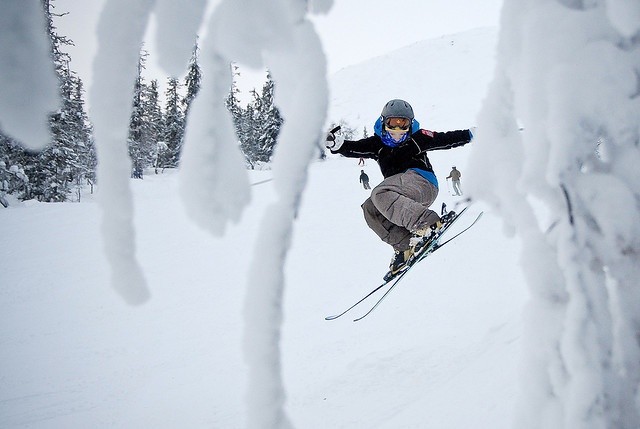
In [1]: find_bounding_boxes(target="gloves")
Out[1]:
[470,124,479,139]
[325,123,345,151]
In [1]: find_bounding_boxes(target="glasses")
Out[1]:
[385,115,412,129]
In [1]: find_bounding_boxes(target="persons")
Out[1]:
[446,166,463,195]
[323,98,477,275]
[358,157,365,166]
[360,170,371,189]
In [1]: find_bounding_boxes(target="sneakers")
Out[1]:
[412,218,442,257]
[389,244,414,275]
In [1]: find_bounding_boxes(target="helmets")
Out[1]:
[381,99,414,130]
